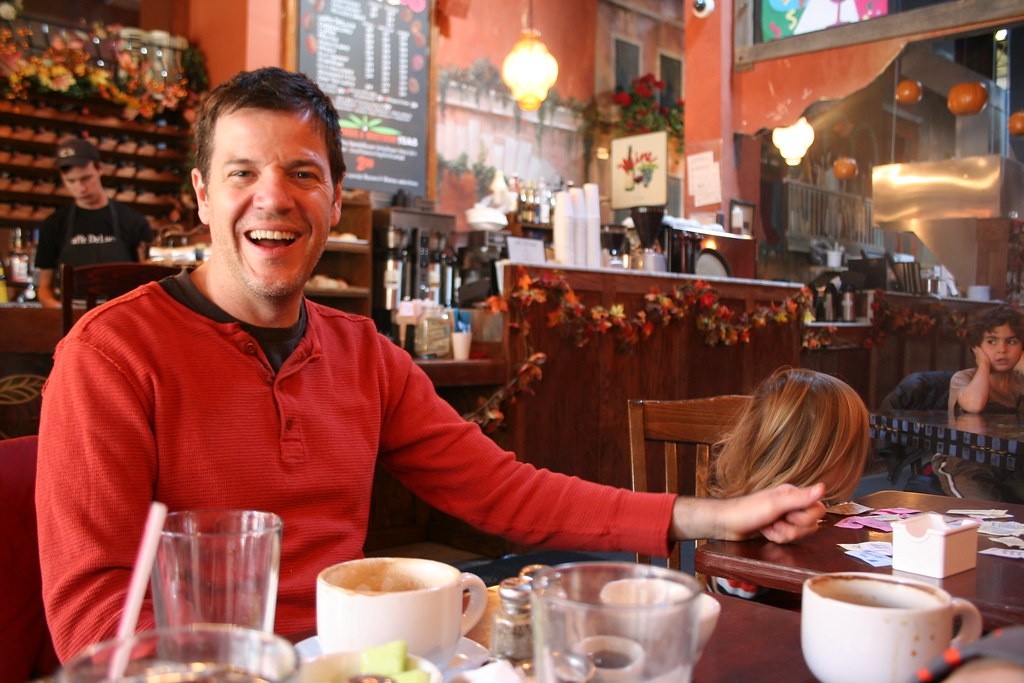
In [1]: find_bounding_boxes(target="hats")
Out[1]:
[55,137,99,168]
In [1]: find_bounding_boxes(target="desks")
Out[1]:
[278,559,821,683]
[694,488,1024,634]
[867,406,1024,475]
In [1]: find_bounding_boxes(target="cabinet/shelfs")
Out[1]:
[0,69,199,251]
[301,189,374,323]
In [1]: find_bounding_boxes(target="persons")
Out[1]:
[35,67,825,671]
[695,368,870,609]
[34,139,149,310]
[932,306,1024,505]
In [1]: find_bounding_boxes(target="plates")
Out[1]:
[295,635,488,683]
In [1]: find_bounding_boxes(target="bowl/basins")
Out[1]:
[601,578,720,662]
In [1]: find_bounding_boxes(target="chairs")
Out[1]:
[59,262,183,340]
[626,394,757,597]
[875,368,958,410]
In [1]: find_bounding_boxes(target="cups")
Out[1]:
[54,622,299,683]
[553,182,602,267]
[534,561,699,682]
[315,557,488,670]
[800,572,983,683]
[827,250,841,268]
[151,510,280,632]
[452,332,472,360]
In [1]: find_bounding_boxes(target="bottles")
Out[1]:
[517,178,554,227]
[519,564,554,580]
[732,206,743,234]
[841,283,855,322]
[384,229,412,310]
[488,575,534,664]
[823,284,836,321]
[0,228,41,303]
[421,231,453,309]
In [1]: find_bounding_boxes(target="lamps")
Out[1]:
[771,113,815,167]
[500,0,558,112]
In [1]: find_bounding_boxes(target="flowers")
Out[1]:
[612,69,687,140]
